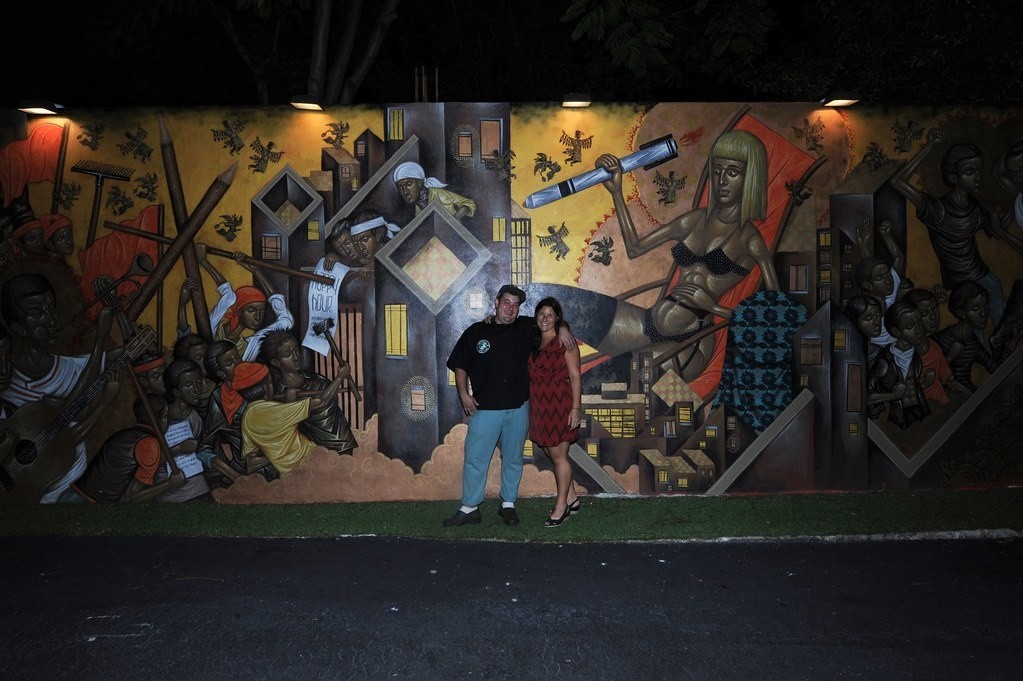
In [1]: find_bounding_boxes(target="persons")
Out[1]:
[443,285,576,526]
[486,297,581,528]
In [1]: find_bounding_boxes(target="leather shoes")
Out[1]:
[498,501,519,526]
[443,507,482,527]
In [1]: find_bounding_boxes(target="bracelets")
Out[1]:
[572,408,581,410]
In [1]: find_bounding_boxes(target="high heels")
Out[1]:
[548,497,580,515]
[543,504,570,528]
